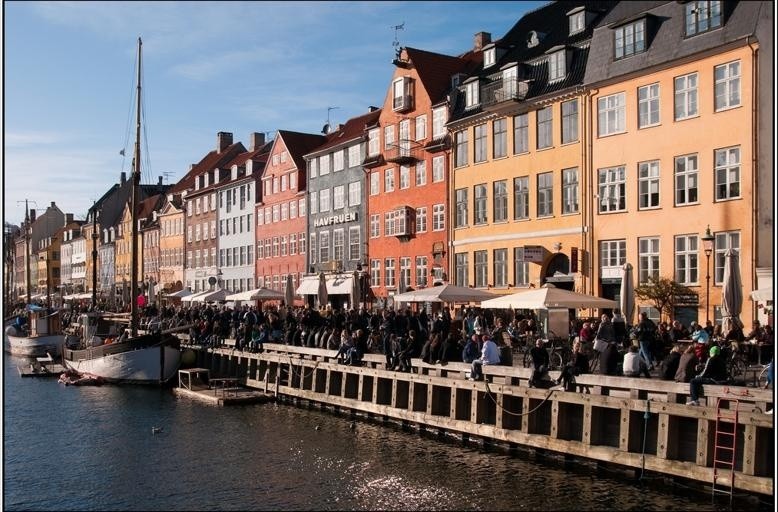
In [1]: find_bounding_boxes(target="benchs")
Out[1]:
[210,384,222,396]
[35,357,56,372]
[221,387,243,398]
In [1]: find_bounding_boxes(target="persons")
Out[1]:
[5,296,773,416]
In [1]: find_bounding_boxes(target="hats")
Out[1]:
[711,346,720,353]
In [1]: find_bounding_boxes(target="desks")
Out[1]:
[177,367,210,390]
[208,376,245,399]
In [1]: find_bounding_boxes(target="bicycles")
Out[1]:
[716,338,748,385]
[757,363,770,390]
[522,335,535,368]
[588,331,609,374]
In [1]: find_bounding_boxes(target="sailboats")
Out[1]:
[5,198,71,360]
[60,37,181,391]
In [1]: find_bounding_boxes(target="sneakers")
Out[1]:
[687,401,699,406]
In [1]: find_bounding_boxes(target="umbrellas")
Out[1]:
[318,269,329,308]
[122,277,128,309]
[480,283,619,338]
[721,246,746,342]
[148,276,155,306]
[285,273,295,307]
[393,285,498,309]
[166,286,302,308]
[619,262,635,327]
[351,270,361,311]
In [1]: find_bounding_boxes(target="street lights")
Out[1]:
[699,224,717,321]
[143,273,149,295]
[359,257,370,310]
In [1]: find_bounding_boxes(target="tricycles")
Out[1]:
[543,330,569,370]
